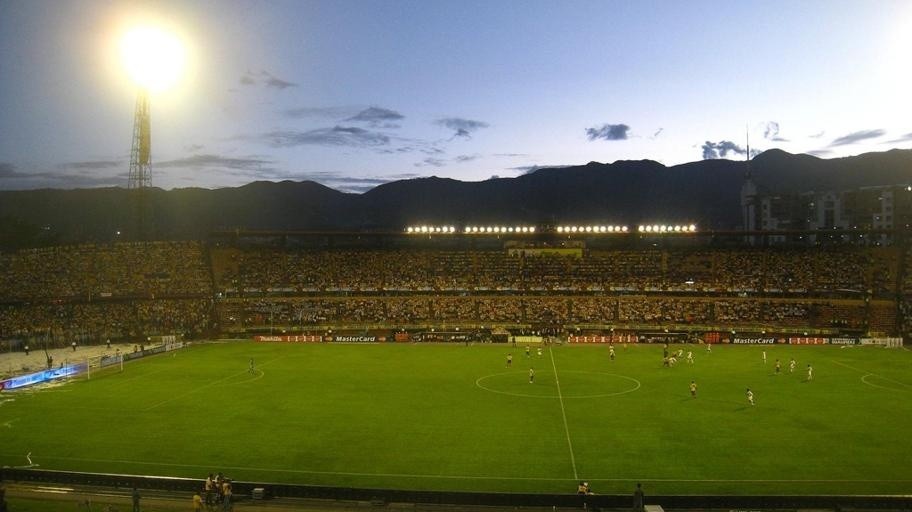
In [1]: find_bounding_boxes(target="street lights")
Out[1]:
[119,28,178,241]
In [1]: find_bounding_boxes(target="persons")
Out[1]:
[744,388,756,406]
[131,485,141,511]
[631,482,645,512]
[688,379,697,399]
[575,480,589,497]
[192,472,234,511]
[26,448,33,465]
[1,234,911,383]
